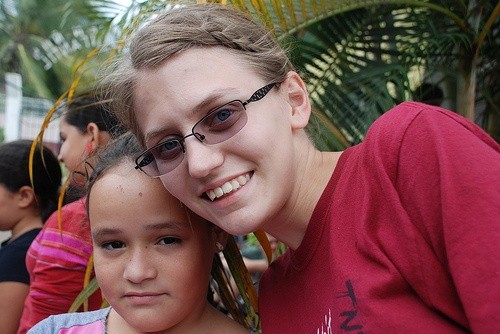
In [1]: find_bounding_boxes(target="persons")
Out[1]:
[15,83,131,333]
[20,132,253,333]
[0,138,62,334]
[91,2,500,333]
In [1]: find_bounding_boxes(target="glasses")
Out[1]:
[134,77,282,179]
